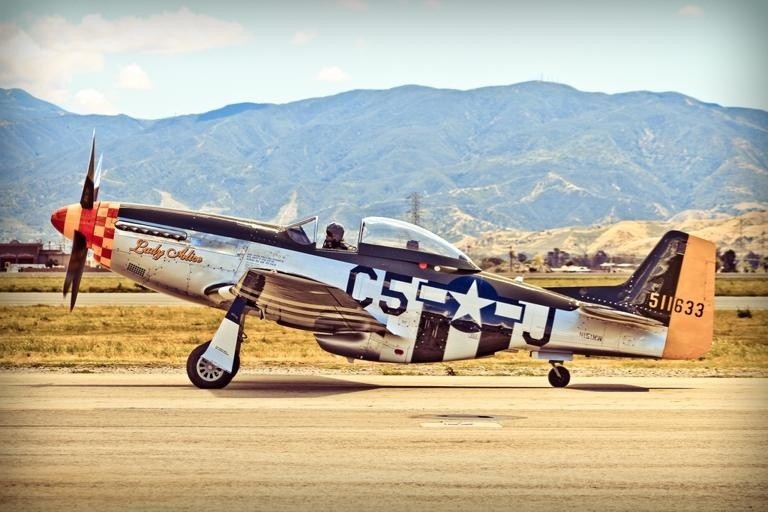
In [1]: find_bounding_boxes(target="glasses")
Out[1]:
[327,231,332,236]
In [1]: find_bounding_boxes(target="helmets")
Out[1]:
[326,223,344,242]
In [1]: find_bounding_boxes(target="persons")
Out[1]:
[323,222,348,250]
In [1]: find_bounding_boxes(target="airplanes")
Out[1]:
[49,123,719,393]
